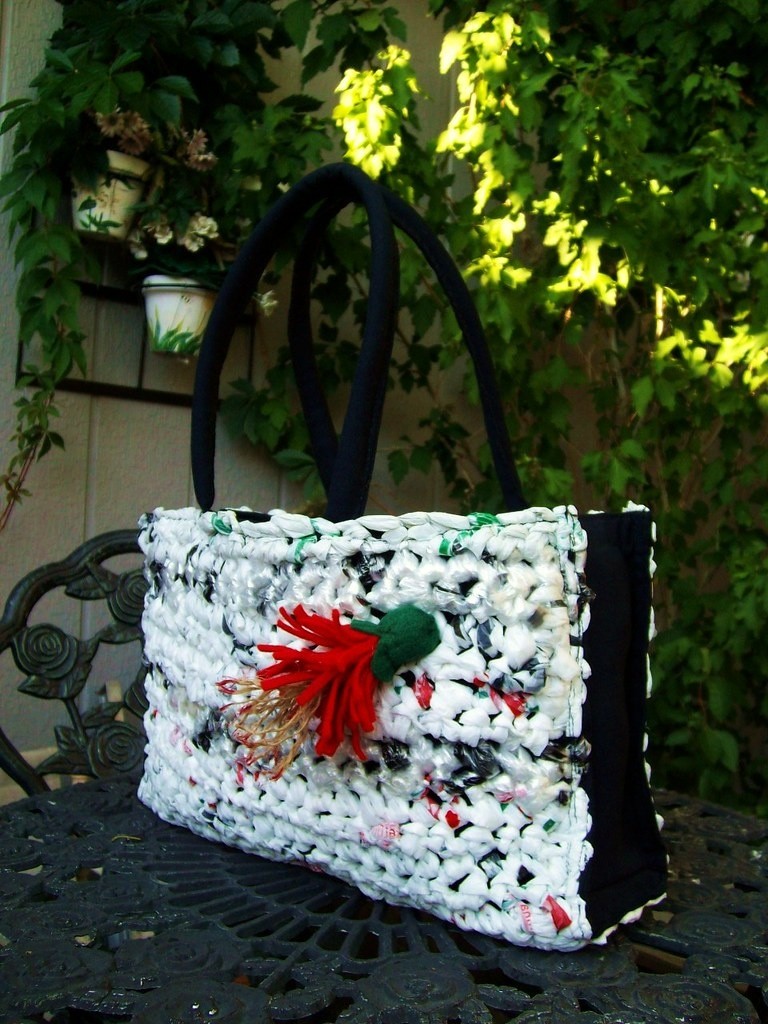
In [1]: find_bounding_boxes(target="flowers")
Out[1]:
[130,214,280,314]
[87,109,217,183]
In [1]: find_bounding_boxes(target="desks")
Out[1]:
[1,762,767,1021]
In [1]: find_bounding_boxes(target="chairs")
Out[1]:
[1,530,153,809]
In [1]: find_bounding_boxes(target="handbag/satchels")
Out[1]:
[138,162,672,954]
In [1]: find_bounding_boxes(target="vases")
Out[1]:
[70,151,171,239]
[141,275,221,354]
